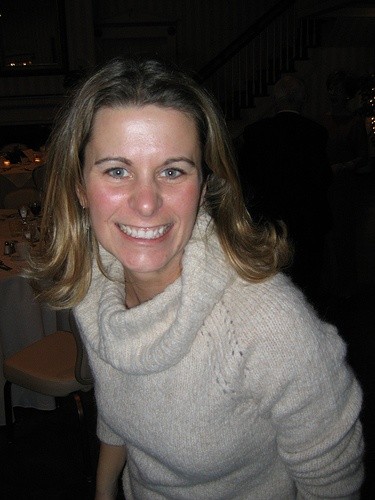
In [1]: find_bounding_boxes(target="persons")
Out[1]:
[232,69,368,312]
[14,62,365,500]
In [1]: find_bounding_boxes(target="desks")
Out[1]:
[1,212,52,270]
[0,149,41,187]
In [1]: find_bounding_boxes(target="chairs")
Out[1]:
[2,165,44,210]
[1,305,94,461]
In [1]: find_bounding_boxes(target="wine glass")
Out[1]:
[17,201,42,252]
